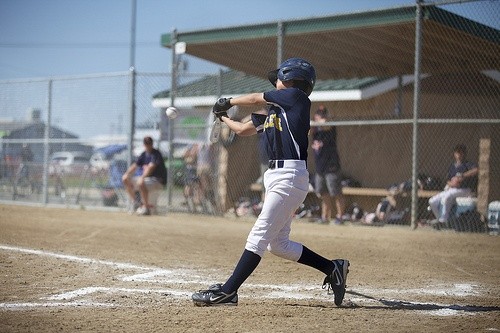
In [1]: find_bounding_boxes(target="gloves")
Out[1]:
[213,98,233,123]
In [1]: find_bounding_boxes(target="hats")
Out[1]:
[315,106,328,114]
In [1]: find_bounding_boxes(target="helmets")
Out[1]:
[268,58,316,97]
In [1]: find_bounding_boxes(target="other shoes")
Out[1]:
[135,203,142,210]
[317,219,330,224]
[335,217,344,224]
[138,208,150,215]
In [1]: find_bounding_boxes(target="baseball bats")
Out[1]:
[210,114,226,144]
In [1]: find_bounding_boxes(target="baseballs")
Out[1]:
[165,108,176,119]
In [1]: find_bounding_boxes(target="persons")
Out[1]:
[122,137,168,216]
[192,58,350,307]
[429,145,478,229]
[311,105,343,225]
[182,137,217,215]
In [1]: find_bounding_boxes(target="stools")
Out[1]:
[133,184,161,214]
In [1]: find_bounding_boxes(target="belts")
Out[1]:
[269,161,284,169]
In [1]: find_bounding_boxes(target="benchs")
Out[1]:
[251,183,472,217]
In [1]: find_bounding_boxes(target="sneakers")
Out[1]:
[192,283,238,306]
[322,259,350,306]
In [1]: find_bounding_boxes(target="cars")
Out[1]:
[48,151,89,176]
